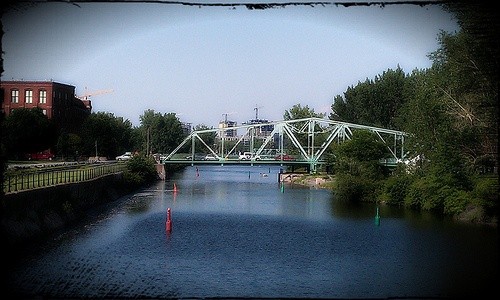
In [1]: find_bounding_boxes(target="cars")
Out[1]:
[28,151,56,161]
[275,153,297,161]
[204,153,223,161]
[185,154,207,161]
[239,150,262,162]
[151,153,169,160]
[116,151,138,160]
